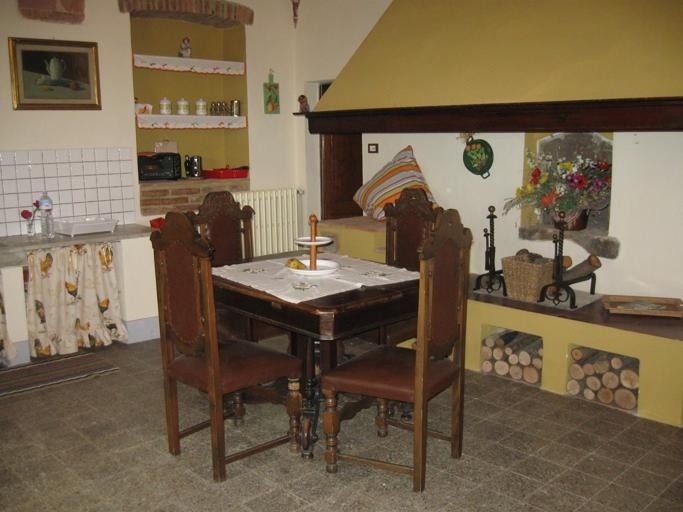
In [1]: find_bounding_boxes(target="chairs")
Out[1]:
[183,189,299,413]
[336,186,444,418]
[150,212,304,484]
[319,208,473,494]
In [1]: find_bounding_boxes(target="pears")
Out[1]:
[287,258,306,269]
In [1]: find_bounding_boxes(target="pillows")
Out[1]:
[352,144,439,222]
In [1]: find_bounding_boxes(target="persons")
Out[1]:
[178,37,192,58]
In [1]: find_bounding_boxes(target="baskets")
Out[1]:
[497,252,556,304]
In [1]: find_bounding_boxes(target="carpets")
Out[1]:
[0,351,121,397]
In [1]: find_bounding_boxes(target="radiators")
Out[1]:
[228,187,300,259]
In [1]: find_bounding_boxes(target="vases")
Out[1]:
[25,219,36,236]
[553,209,590,231]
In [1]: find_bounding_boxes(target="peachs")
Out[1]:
[268,102,275,113]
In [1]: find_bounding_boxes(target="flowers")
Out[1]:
[502,143,611,223]
[20,200,41,219]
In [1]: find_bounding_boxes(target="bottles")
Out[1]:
[158,97,171,115]
[184,155,190,177]
[175,97,190,115]
[195,98,207,116]
[38,191,54,241]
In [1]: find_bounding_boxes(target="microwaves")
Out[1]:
[136,152,182,182]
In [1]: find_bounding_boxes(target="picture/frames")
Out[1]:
[8,36,101,111]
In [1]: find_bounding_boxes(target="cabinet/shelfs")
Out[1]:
[133,52,247,130]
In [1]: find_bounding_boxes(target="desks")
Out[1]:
[1,223,155,369]
